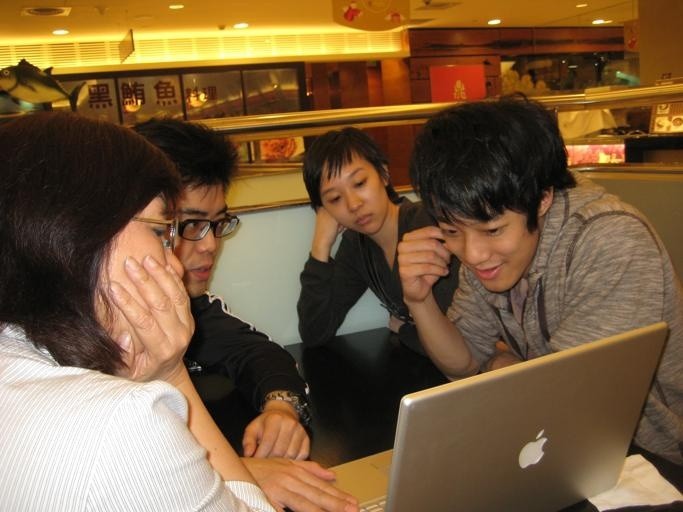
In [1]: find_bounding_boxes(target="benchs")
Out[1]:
[580,173,682,283]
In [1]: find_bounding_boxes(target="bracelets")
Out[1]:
[260,390,313,427]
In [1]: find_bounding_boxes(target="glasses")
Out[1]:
[179,214,239,241]
[132,217,178,251]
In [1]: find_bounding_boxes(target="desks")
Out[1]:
[189,328,682,512]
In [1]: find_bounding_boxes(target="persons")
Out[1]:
[132,116,358,512]
[295,126,463,357]
[395,95,682,467]
[0,110,274,512]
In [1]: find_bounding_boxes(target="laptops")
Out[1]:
[354,319,671,512]
